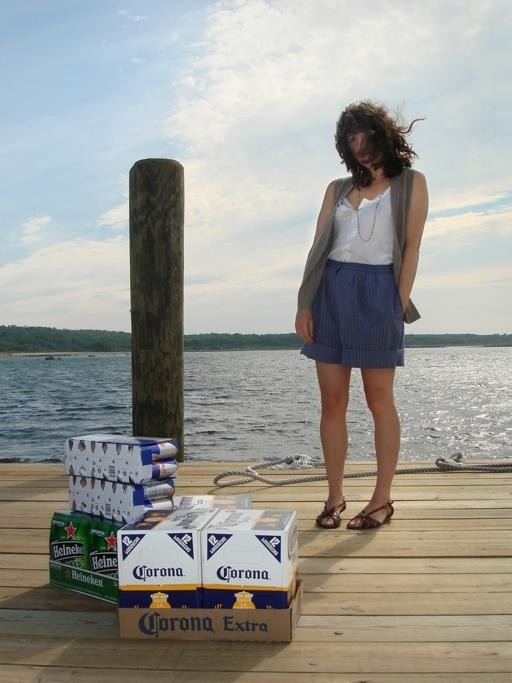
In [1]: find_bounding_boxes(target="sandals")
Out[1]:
[347,500,394,529]
[316,501,346,529]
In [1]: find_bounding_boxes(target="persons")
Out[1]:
[294,100,432,530]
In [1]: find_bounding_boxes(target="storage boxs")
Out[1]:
[46,492,303,644]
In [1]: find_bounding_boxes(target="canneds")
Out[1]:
[143,458,178,482]
[145,499,174,516]
[141,441,178,465]
[144,480,175,500]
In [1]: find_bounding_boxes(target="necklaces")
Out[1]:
[354,176,388,239]
